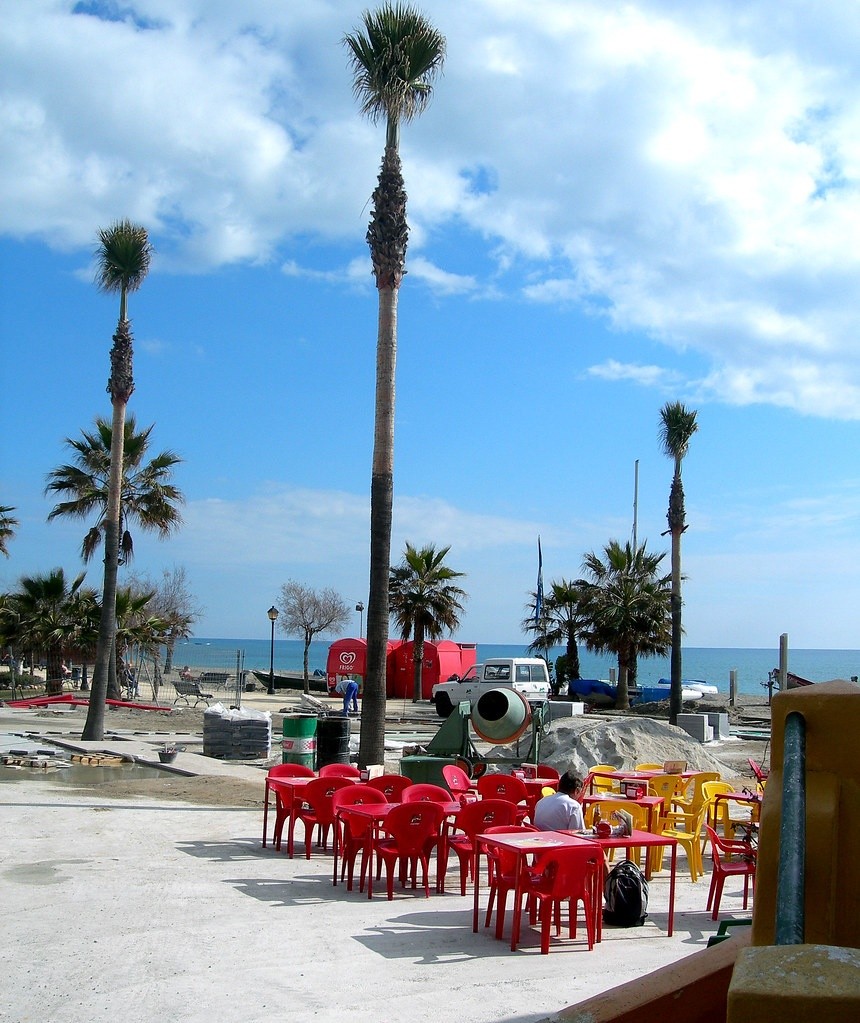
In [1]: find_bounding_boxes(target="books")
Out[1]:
[615,808,633,837]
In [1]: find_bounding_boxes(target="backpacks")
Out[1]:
[603,858,648,925]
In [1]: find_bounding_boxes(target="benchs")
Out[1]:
[61,668,72,691]
[170,681,213,708]
[117,672,138,700]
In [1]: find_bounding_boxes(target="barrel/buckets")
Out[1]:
[246,684,255,691]
[282,715,318,771]
[316,717,351,771]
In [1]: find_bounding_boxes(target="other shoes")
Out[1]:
[135,692,142,697]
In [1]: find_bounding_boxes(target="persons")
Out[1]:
[2,653,14,668]
[123,663,143,697]
[61,657,72,679]
[534,769,587,833]
[329,679,358,717]
[179,666,192,682]
[16,652,25,675]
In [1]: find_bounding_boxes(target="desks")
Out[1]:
[473,831,601,952]
[438,801,468,872]
[589,772,660,809]
[581,792,665,879]
[643,769,703,827]
[263,777,318,856]
[557,828,678,937]
[333,803,401,900]
[712,791,764,862]
[341,777,369,788]
[514,778,559,806]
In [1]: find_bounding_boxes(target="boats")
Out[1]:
[772,669,815,689]
[564,676,719,707]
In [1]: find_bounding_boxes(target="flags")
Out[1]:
[534,540,544,624]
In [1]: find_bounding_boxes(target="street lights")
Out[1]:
[355,600,365,641]
[265,606,279,694]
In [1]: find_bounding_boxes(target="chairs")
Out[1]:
[269,760,768,955]
[503,672,509,679]
[486,671,496,679]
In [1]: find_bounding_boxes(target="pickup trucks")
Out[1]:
[430,657,551,717]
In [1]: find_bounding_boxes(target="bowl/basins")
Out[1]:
[158,751,178,763]
